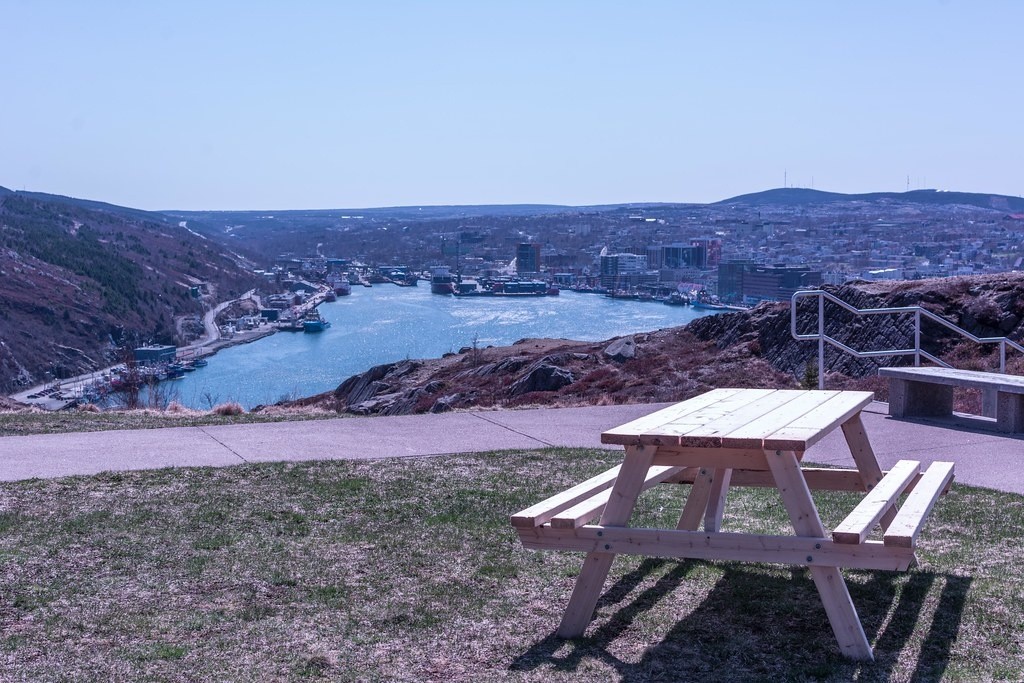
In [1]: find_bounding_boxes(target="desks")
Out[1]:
[558,389,918,662]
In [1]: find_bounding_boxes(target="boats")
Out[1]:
[143,357,208,384]
[324,277,351,301]
[568,275,728,310]
[275,298,331,331]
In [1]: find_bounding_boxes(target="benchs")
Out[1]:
[878,366,1024,433]
[834,459,956,570]
[509,463,688,549]
